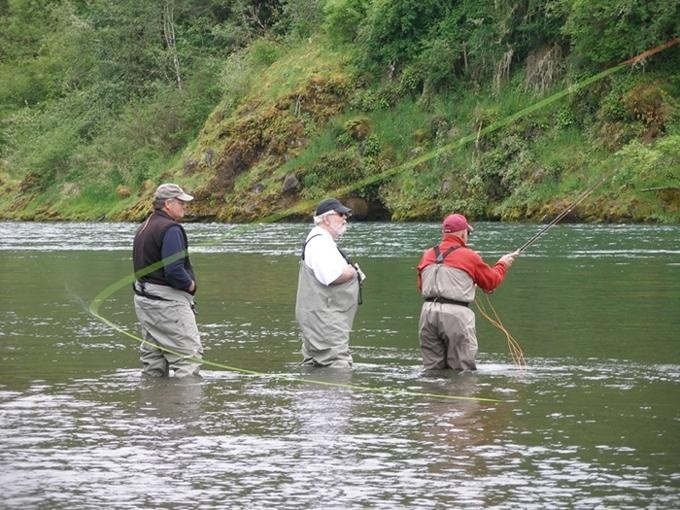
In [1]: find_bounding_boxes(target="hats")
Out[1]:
[155,183,195,202]
[443,213,474,232]
[316,198,353,214]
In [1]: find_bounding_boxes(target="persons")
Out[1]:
[132,182,204,380]
[416,213,521,373]
[294,197,362,370]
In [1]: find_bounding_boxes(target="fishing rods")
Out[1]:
[515,131,654,254]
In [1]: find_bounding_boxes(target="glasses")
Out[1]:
[326,211,348,218]
